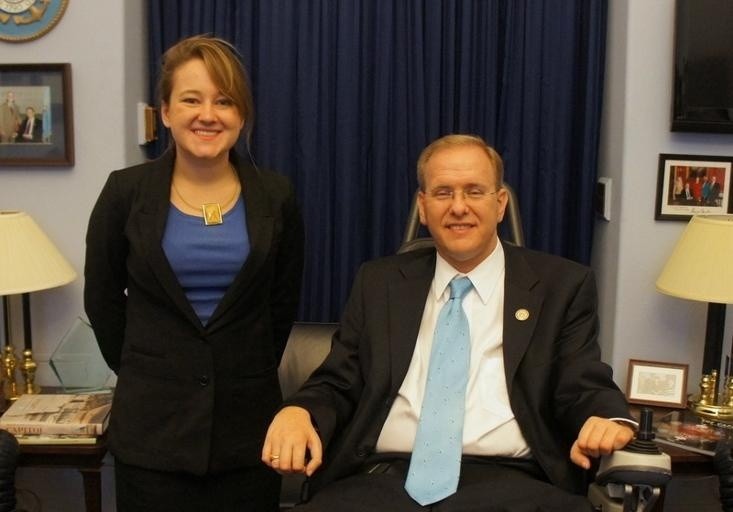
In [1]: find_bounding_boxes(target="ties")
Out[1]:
[26,121,31,135]
[404,275,474,507]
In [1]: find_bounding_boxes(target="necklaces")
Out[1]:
[174,164,239,226]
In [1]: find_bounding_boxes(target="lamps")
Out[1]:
[0,212,76,400]
[657,216,733,425]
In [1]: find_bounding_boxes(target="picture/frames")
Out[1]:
[0,62,76,168]
[655,153,732,221]
[625,359,690,413]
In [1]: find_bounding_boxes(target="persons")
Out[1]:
[84,37,306,512]
[1,92,43,143]
[261,136,639,512]
[667,165,725,206]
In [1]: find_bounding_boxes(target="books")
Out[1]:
[650,410,733,456]
[0,393,114,443]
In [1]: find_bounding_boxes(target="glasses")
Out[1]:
[422,185,497,200]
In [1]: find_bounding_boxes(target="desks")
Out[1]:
[670,414,733,512]
[1,386,112,512]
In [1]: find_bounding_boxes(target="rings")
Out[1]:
[270,454,279,461]
